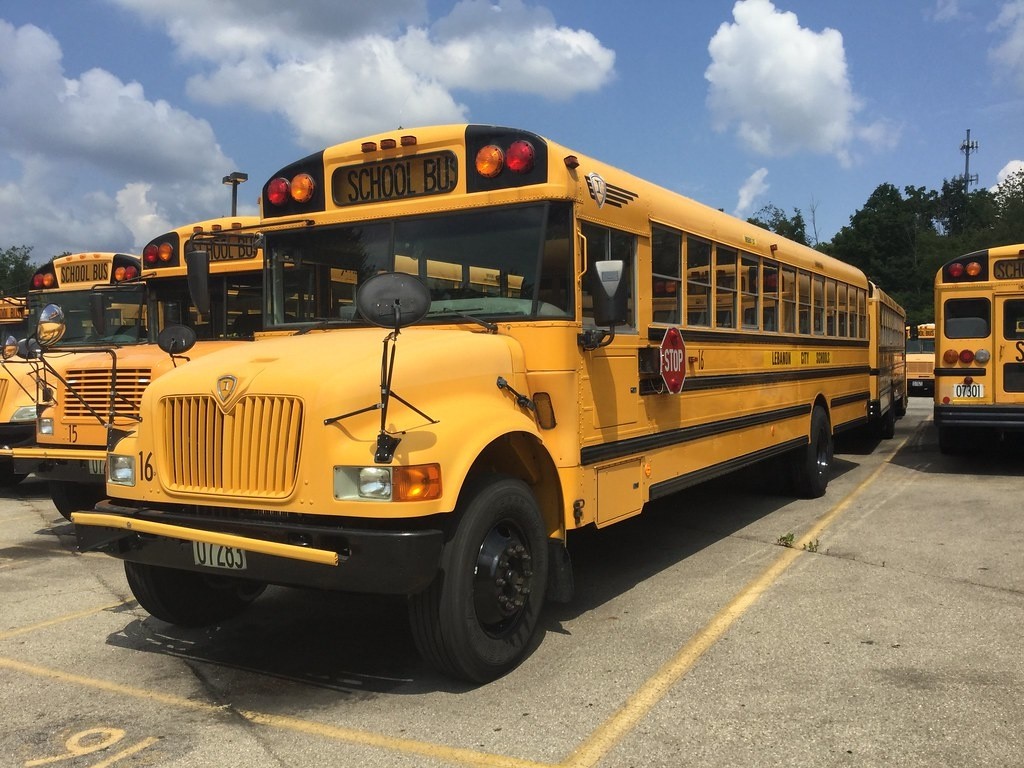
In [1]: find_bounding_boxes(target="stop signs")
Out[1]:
[660,325,686,395]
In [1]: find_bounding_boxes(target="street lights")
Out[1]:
[222,172,248,217]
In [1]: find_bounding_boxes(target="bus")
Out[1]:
[933,243,1024,458]
[0,217,524,522]
[905,324,935,397]
[869,281,919,439]
[71,125,870,683]
